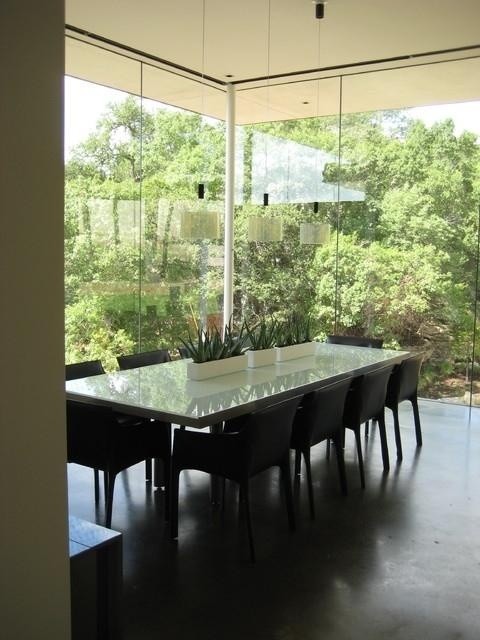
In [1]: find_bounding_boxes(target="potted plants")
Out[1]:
[175,313,262,380]
[245,316,282,368]
[271,310,316,362]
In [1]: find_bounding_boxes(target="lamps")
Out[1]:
[299,3,331,244]
[180,0,219,239]
[247,0,283,241]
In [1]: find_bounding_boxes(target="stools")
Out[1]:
[68,518,121,640]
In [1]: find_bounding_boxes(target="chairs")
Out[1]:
[326,335,384,422]
[65,359,151,479]
[115,349,185,430]
[364,351,424,463]
[291,376,352,521]
[175,341,213,359]
[326,362,395,489]
[65,400,162,528]
[169,393,305,566]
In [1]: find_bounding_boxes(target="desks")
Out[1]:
[66,340,411,505]
